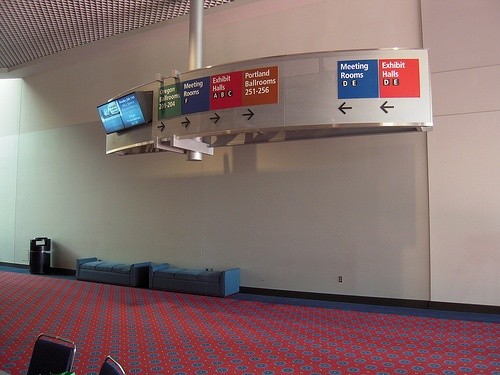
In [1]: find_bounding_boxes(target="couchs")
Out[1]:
[148,263,239,296]
[76,257,151,288]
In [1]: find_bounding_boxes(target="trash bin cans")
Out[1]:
[29,237,53,275]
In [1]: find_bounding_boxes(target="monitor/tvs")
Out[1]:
[96,91,152,135]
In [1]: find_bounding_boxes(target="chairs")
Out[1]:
[26,333,77,375]
[100,355,126,375]
